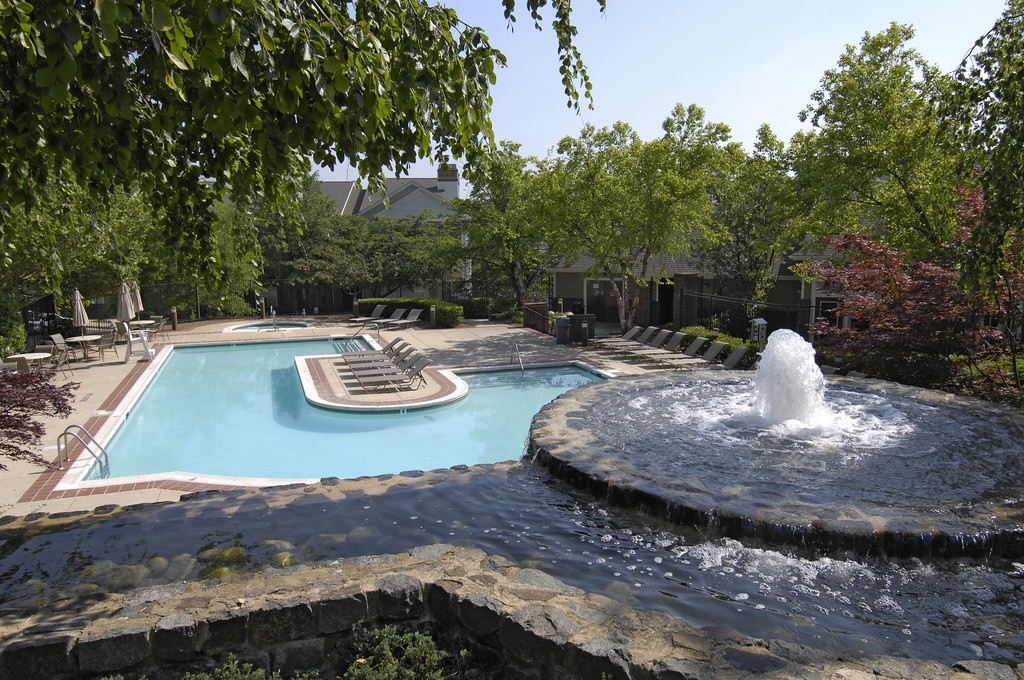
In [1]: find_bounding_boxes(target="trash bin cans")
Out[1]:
[555,317,571,344]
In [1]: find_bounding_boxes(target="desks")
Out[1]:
[127,319,156,340]
[6,353,51,375]
[65,334,102,363]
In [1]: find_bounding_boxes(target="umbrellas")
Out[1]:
[117,278,136,332]
[72,287,91,338]
[130,279,145,314]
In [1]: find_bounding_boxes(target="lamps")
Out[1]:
[582,320,588,328]
[558,297,563,304]
[430,305,436,311]
[171,306,176,313]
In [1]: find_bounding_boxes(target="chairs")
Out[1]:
[368,307,405,331]
[0,313,170,381]
[588,325,750,373]
[348,304,387,328]
[388,309,425,330]
[341,337,434,395]
